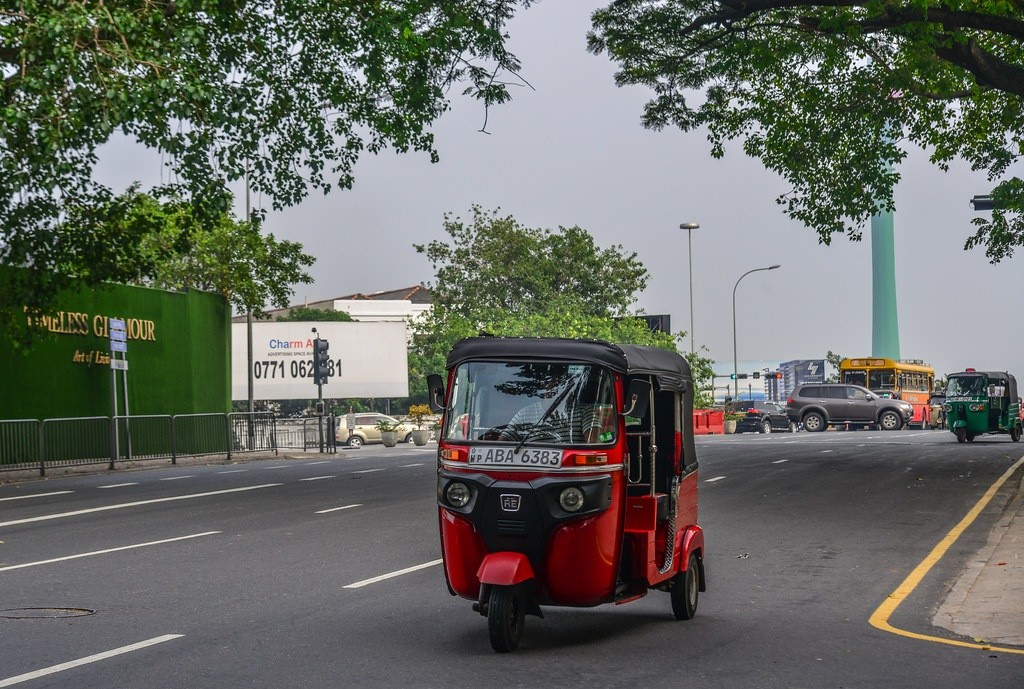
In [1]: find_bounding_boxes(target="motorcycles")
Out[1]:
[426,329,706,654]
[929,368,1024,444]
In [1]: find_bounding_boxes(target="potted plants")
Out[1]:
[409,404,432,445]
[373,419,399,447]
[724,411,739,433]
[433,420,442,442]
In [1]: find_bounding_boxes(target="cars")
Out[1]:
[731,400,799,435]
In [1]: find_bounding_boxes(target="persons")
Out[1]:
[1017,397,1024,420]
[965,384,986,396]
[497,377,605,444]
[848,373,900,399]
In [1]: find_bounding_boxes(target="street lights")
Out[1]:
[680,222,701,353]
[733,264,782,400]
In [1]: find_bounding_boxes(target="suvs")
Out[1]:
[335,412,419,450]
[786,382,914,433]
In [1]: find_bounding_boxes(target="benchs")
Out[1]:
[1001,398,1009,424]
[627,424,671,522]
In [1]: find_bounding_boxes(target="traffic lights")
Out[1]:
[753,372,761,379]
[313,339,331,384]
[731,373,747,380]
[765,372,782,380]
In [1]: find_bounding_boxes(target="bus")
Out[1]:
[838,357,935,431]
